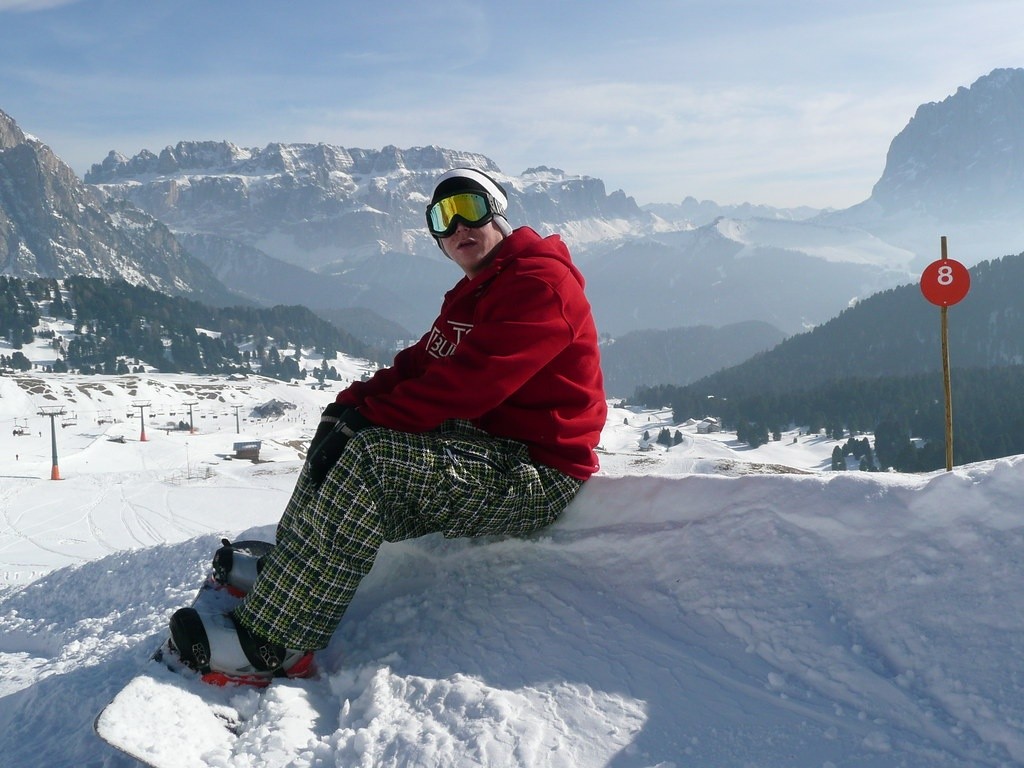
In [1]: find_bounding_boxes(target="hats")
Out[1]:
[425,166,510,259]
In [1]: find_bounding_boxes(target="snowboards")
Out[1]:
[93,540,281,768]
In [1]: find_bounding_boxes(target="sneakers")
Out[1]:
[216,538,265,595]
[163,608,313,686]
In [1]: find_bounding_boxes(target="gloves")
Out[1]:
[307,403,342,468]
[310,408,370,487]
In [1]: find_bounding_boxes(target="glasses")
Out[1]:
[425,194,506,241]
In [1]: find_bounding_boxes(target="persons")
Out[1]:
[170,169,607,678]
